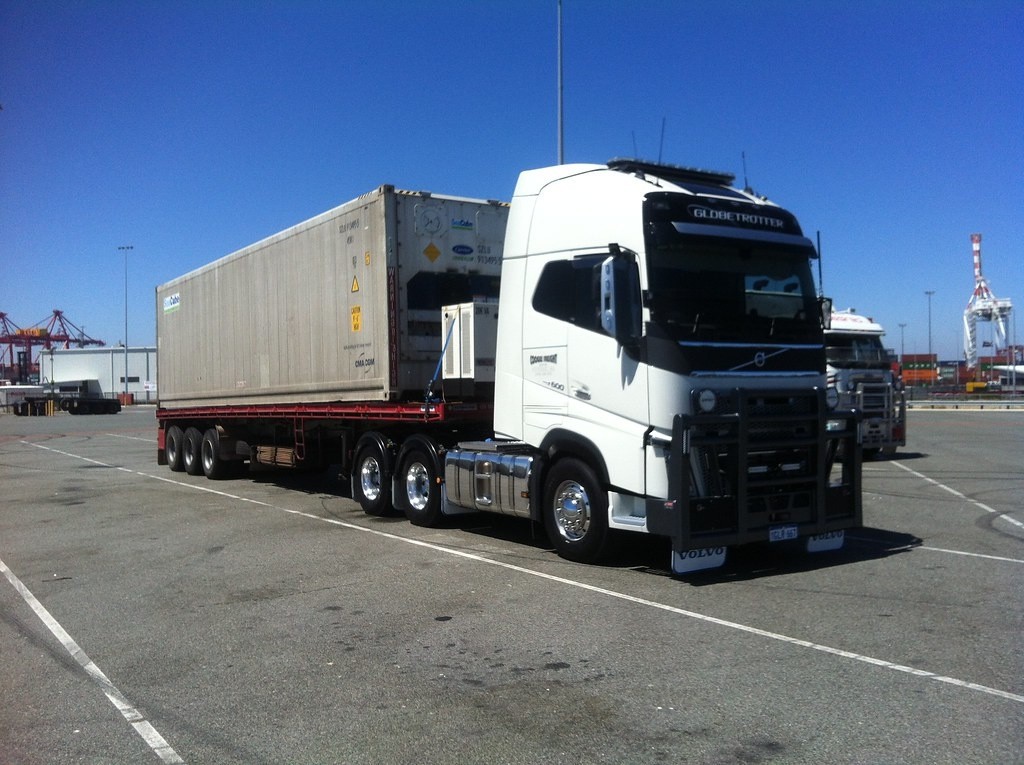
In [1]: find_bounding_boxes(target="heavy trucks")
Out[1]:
[823,305,907,460]
[154,157,866,575]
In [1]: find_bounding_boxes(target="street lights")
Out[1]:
[117,246,135,407]
